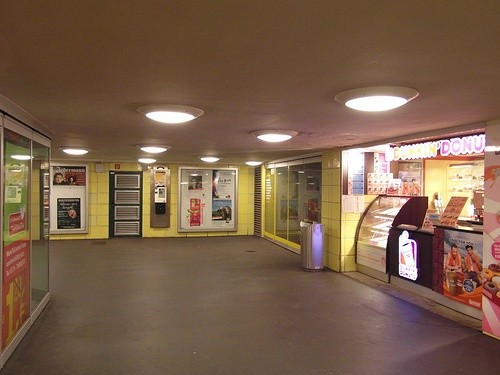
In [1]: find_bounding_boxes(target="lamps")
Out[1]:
[245,161,262,166]
[136,104,204,124]
[63,148,89,156]
[138,158,156,164]
[136,145,172,154]
[250,129,298,143]
[200,156,220,163]
[333,86,419,112]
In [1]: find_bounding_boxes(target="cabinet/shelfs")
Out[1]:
[355,194,428,274]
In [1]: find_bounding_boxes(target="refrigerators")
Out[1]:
[355,194,428,283]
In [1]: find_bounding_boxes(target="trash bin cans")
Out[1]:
[298,219,326,273]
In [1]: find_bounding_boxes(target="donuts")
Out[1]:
[482,269,499,294]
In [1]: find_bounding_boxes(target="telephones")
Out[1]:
[154,186,166,203]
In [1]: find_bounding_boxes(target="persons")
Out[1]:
[188,178,201,190]
[445,245,483,288]
[53,173,77,185]
[401,178,420,196]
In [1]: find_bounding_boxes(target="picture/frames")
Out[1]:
[50,164,89,235]
[177,167,239,233]
